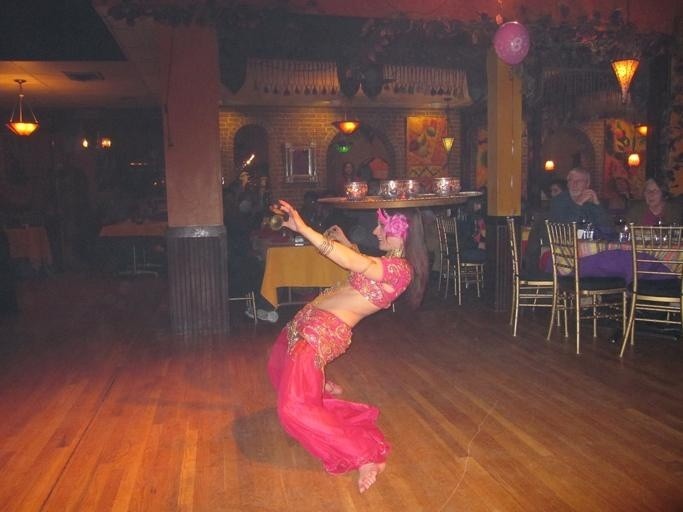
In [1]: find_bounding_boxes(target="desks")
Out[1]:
[315,190,485,311]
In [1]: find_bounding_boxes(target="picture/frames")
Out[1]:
[283,140,318,184]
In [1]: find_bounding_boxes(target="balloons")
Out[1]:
[494,19,531,67]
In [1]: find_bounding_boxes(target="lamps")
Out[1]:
[441,98,455,153]
[610,58,640,105]
[3,79,42,137]
[333,119,360,135]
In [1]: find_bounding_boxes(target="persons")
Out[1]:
[521,168,681,316]
[222,161,277,322]
[336,163,359,195]
[266,198,432,496]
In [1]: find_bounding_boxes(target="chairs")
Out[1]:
[434,211,682,357]
